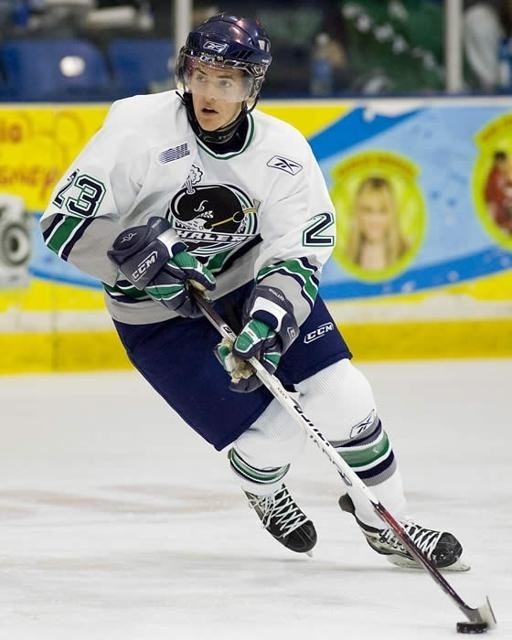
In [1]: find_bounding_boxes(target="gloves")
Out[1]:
[213,284,299,393]
[107,216,216,319]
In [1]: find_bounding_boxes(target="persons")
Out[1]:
[39,14,462,567]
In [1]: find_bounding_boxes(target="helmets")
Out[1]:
[175,13,271,102]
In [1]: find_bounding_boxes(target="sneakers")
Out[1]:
[339,494,463,568]
[242,484,317,553]
